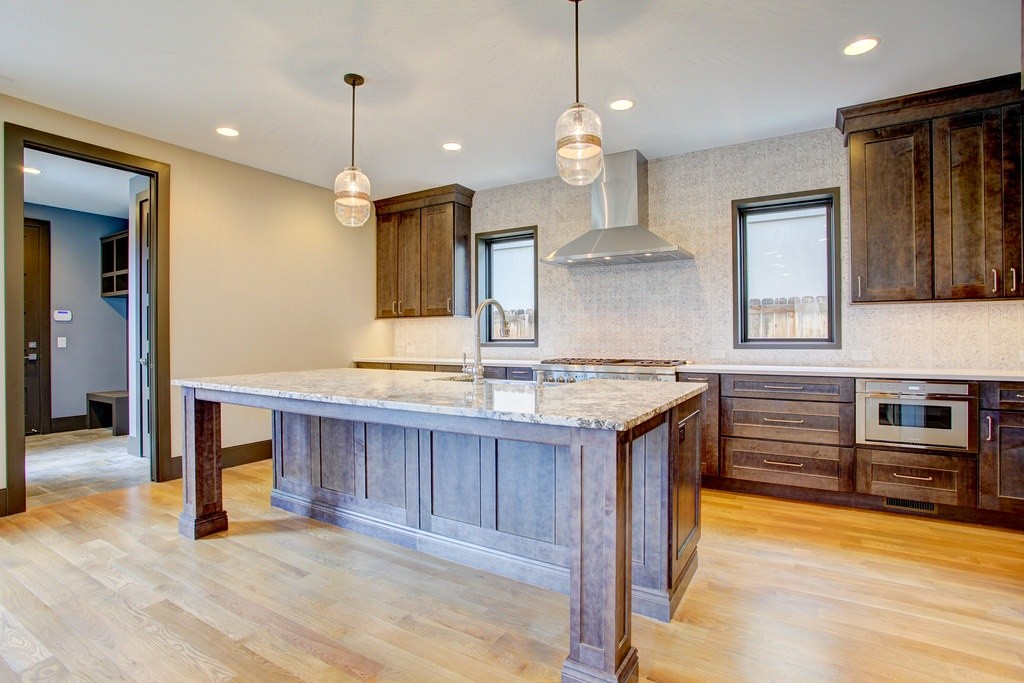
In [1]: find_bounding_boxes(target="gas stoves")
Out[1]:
[542,357,686,367]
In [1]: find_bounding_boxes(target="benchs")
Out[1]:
[85,390,129,436]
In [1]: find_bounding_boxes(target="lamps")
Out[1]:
[555,0,603,185]
[334,72,371,227]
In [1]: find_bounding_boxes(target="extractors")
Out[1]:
[540,149,694,269]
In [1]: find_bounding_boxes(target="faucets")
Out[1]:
[462,299,510,384]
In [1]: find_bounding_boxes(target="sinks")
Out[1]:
[431,375,564,388]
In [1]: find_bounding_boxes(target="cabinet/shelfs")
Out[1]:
[98,229,128,299]
[372,183,475,319]
[976,381,1024,530]
[835,72,1024,304]
[676,372,856,506]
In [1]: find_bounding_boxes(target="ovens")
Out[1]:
[855,379,968,453]
